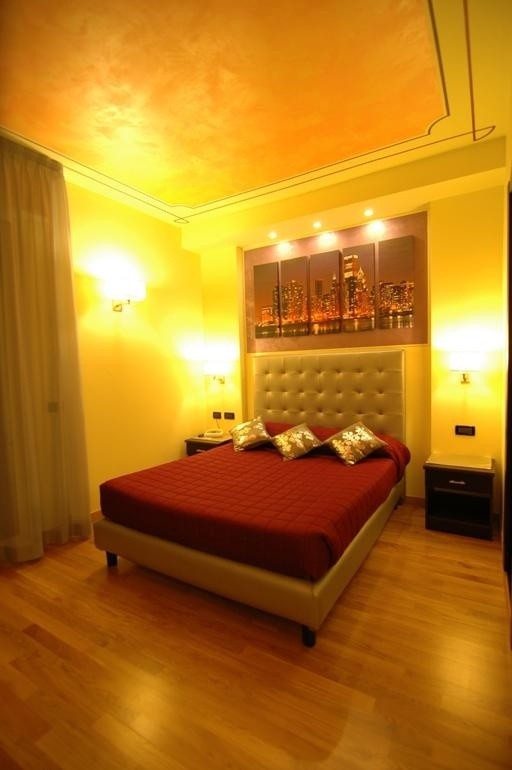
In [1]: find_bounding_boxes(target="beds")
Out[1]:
[92,348,410,648]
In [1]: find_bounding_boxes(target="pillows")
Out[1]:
[271,423,324,462]
[229,416,272,453]
[323,421,390,467]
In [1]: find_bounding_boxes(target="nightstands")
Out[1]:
[423,459,495,541]
[184,438,232,456]
